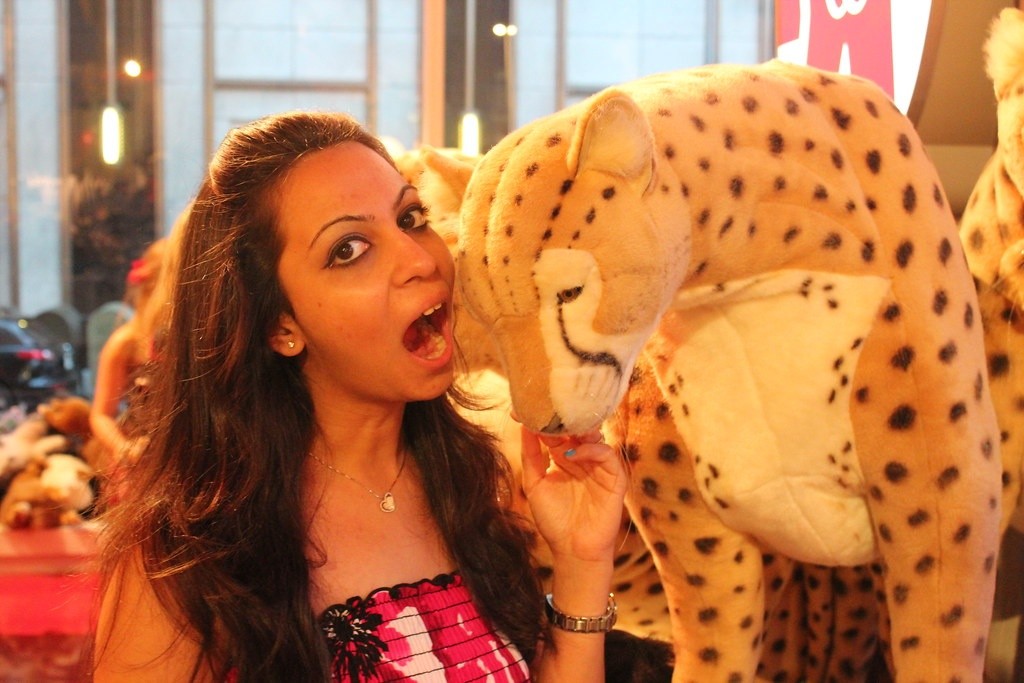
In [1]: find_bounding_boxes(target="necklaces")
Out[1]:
[309,445,408,512]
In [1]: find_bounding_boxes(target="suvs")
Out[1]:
[1,313,80,414]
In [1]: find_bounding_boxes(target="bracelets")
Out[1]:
[545,593,618,632]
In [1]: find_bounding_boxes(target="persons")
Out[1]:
[93,111,628,683]
[89,238,166,464]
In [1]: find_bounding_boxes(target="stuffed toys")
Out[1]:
[0,398,110,526]
[398,8,1024,683]
